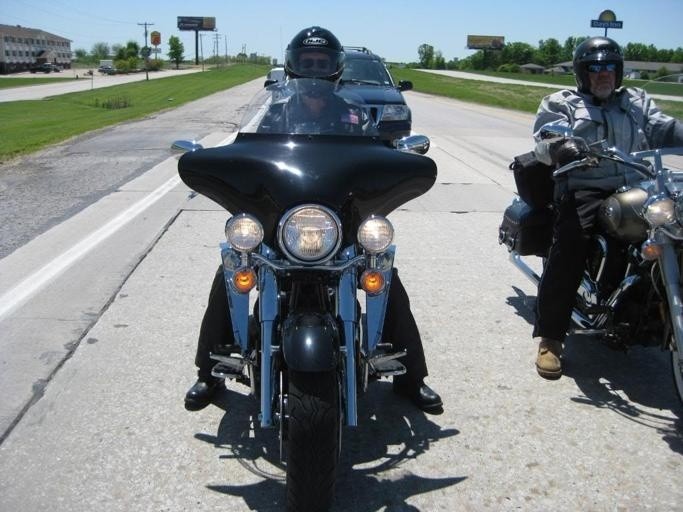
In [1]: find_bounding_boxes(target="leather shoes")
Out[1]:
[392,375,443,410]
[185,368,225,409]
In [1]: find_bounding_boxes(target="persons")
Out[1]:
[533,37,683,377]
[183,26,443,411]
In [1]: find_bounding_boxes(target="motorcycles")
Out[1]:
[494,127,682,411]
[166,76,438,511]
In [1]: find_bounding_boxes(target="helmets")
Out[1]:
[573,36,624,92]
[285,26,345,82]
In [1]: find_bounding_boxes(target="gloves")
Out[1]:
[553,137,583,165]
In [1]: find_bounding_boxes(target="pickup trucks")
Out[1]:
[30,66,51,74]
[42,63,63,72]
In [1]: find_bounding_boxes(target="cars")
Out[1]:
[263,45,414,144]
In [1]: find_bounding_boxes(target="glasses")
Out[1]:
[584,63,618,75]
[299,56,331,71]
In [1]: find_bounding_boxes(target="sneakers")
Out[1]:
[537,337,563,379]
